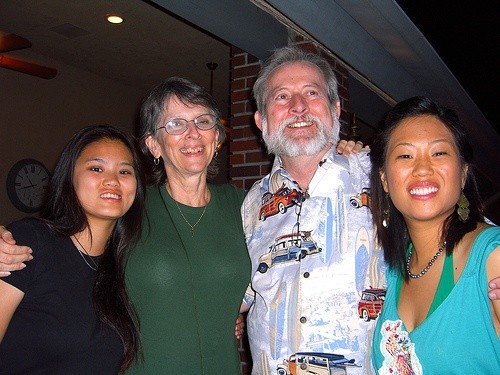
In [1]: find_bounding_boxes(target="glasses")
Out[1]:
[154,114,219,135]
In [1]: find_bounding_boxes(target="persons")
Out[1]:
[0,76,370,375]
[240,46,497,375]
[371,95,500,375]
[0,125,244,375]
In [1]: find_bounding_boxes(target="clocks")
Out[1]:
[5,158,52,214]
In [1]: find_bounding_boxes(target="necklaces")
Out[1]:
[407,240,446,278]
[175,200,207,231]
[73,235,98,271]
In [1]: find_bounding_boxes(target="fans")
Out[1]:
[0,29,58,80]
